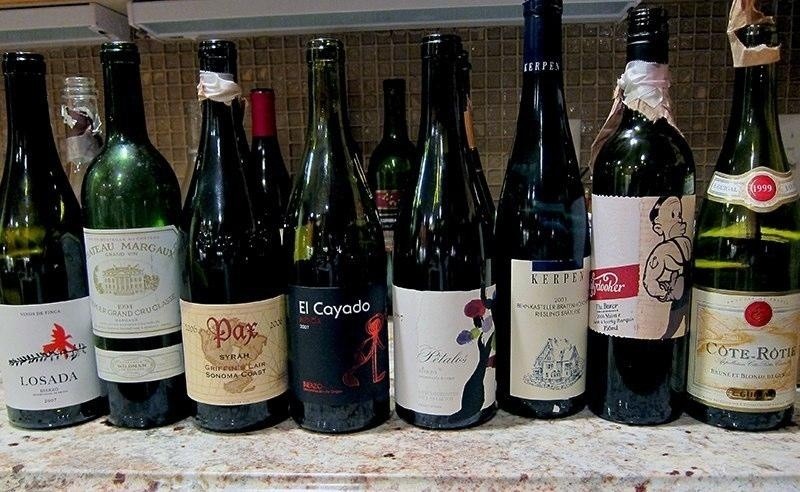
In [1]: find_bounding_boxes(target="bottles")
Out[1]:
[286,37,391,432]
[685,24,800,428]
[84,41,185,431]
[369,36,496,432]
[497,1,586,419]
[37,0,602,255]
[1,50,105,429]
[588,9,696,422]
[176,37,292,435]
